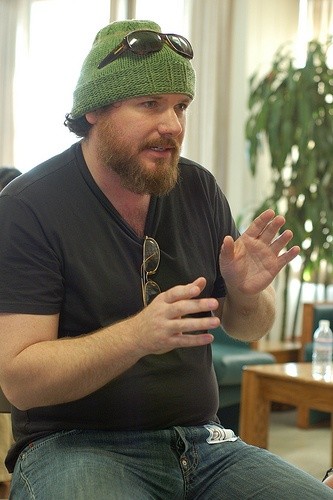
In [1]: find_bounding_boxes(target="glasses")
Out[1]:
[98,29,195,71]
[139,234,161,309]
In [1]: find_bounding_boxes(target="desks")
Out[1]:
[238,363,333,470]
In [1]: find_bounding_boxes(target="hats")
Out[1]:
[70,17,197,120]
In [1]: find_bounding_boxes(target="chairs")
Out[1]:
[208,327,276,409]
[299,304,333,362]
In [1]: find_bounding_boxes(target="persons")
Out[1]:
[0,20,333,500]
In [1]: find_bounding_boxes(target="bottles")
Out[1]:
[312,320,333,383]
